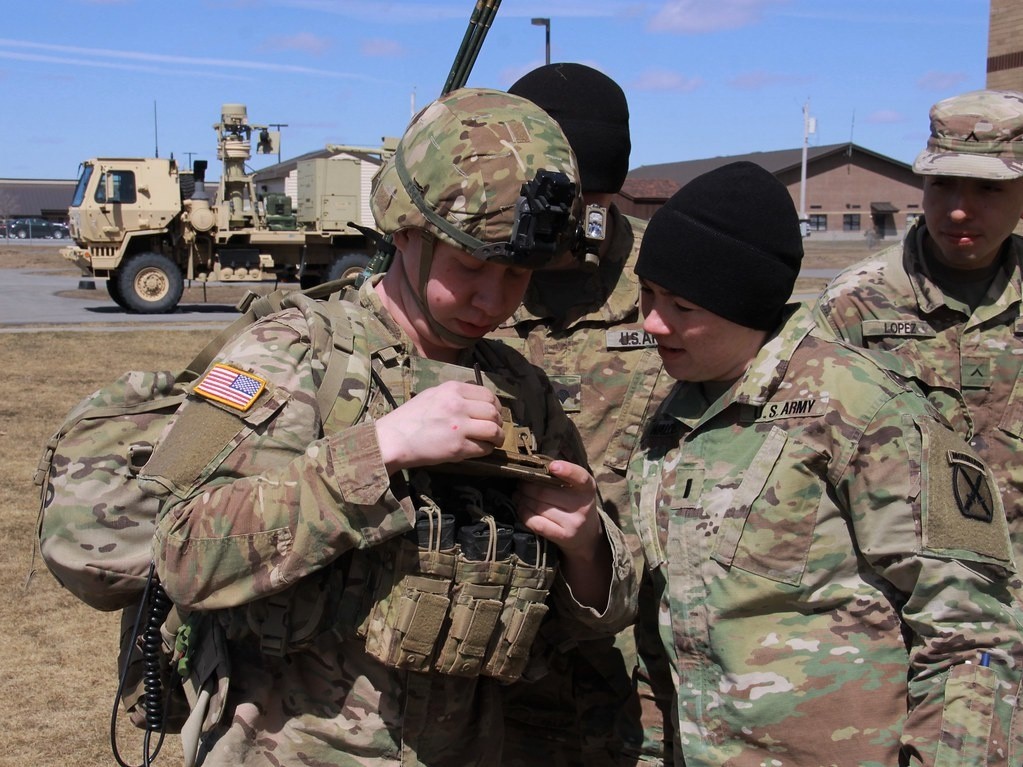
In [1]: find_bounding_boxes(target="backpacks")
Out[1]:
[22,288,371,611]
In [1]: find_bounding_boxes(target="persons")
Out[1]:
[139,88,638,767]
[628,160,1022,767]
[482,63,678,767]
[808,87,1023,599]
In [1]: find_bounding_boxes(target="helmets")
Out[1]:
[369,88,583,261]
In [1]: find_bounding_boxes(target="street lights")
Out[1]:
[532,18,551,64]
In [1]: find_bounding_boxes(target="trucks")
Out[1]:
[59,105,402,315]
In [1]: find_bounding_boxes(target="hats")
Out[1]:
[507,62,631,194]
[633,161,804,331]
[912,90,1023,180]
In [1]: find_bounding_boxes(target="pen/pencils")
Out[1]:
[475,362,484,386]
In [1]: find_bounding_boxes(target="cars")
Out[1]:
[0,218,69,239]
[799,215,811,236]
